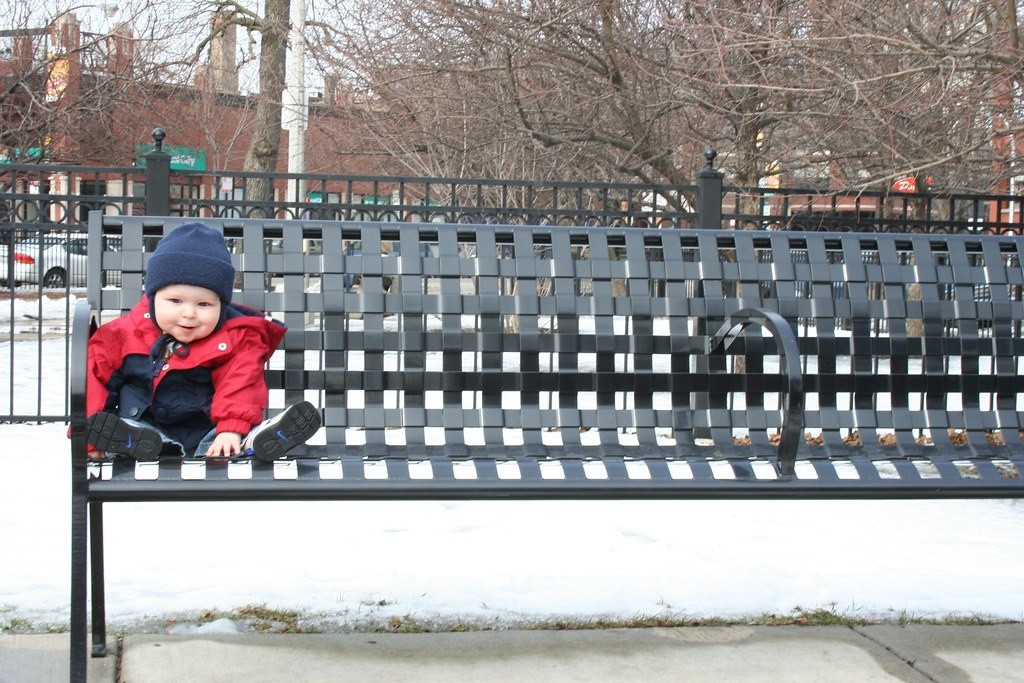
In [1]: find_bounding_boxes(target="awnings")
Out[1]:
[363,194,390,206]
[307,193,341,205]
[0,148,50,165]
[423,200,441,207]
[137,143,206,172]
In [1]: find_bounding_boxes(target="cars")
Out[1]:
[0,233,144,288]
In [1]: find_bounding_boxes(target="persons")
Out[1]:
[68,223,321,464]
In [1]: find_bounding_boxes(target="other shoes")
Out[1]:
[250,401,320,463]
[87,412,163,463]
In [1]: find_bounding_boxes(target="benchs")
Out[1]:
[69,211,1024,683]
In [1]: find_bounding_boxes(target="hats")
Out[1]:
[145,223,234,305]
